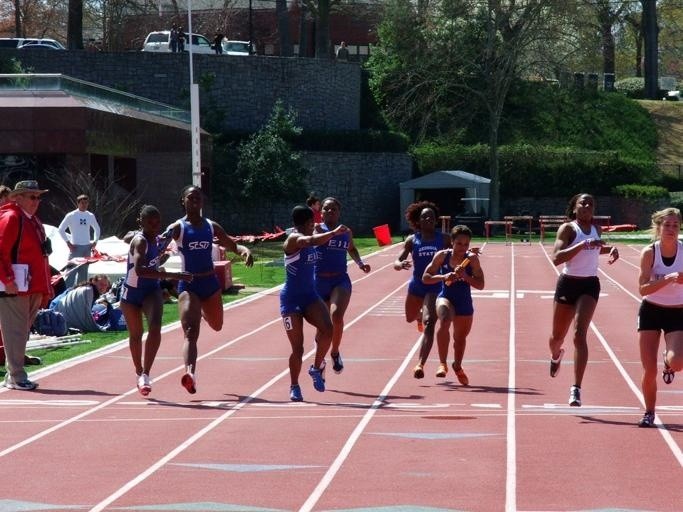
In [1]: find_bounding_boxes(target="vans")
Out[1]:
[138,30,216,53]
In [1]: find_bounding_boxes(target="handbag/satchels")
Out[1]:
[34,307,67,336]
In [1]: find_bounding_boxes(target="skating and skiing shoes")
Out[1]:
[550,349,565,377]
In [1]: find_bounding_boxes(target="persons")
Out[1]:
[393,201,483,379]
[156,184,254,395]
[0,179,53,391]
[421,225,485,385]
[548,192,619,407]
[120,204,193,397]
[278,192,371,402]
[0,184,180,367]
[637,207,683,428]
[168,24,350,63]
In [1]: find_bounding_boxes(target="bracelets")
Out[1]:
[585,239,590,250]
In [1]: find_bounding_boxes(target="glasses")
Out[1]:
[30,195,40,200]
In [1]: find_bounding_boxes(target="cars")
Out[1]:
[0,38,63,47]
[222,40,256,56]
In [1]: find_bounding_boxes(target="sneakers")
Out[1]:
[25,355,41,365]
[3,373,39,390]
[452,362,469,385]
[414,364,424,379]
[662,351,674,384]
[309,359,327,392]
[436,363,448,377]
[331,351,343,375]
[182,364,196,394]
[569,385,582,406]
[136,370,151,396]
[289,384,303,401]
[418,322,424,332]
[638,410,655,427]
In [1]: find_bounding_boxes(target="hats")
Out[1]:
[8,180,49,201]
